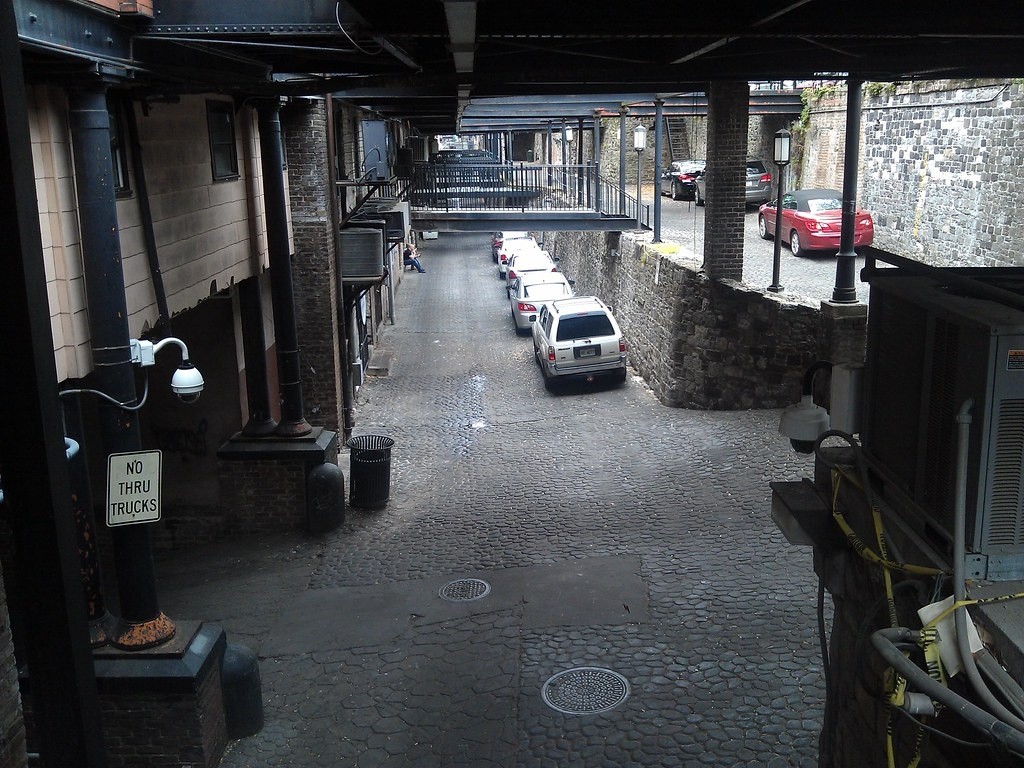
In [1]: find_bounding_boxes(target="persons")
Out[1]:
[403,244,426,273]
[403,243,417,270]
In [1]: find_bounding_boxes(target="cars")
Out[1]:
[661,159,707,201]
[692,156,772,209]
[758,188,874,257]
[495,237,542,278]
[506,271,576,335]
[501,249,561,299]
[489,231,528,261]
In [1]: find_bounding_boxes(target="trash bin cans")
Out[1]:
[306,463,345,527]
[219,643,265,740]
[345,434,395,509]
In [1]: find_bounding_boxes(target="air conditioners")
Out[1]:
[407,134,429,163]
[340,200,409,277]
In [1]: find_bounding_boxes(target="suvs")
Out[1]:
[529,295,628,393]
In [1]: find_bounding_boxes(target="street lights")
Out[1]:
[633,121,645,234]
[565,126,574,207]
[766,125,792,292]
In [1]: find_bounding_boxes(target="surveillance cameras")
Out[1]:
[171,365,204,404]
[778,405,831,455]
